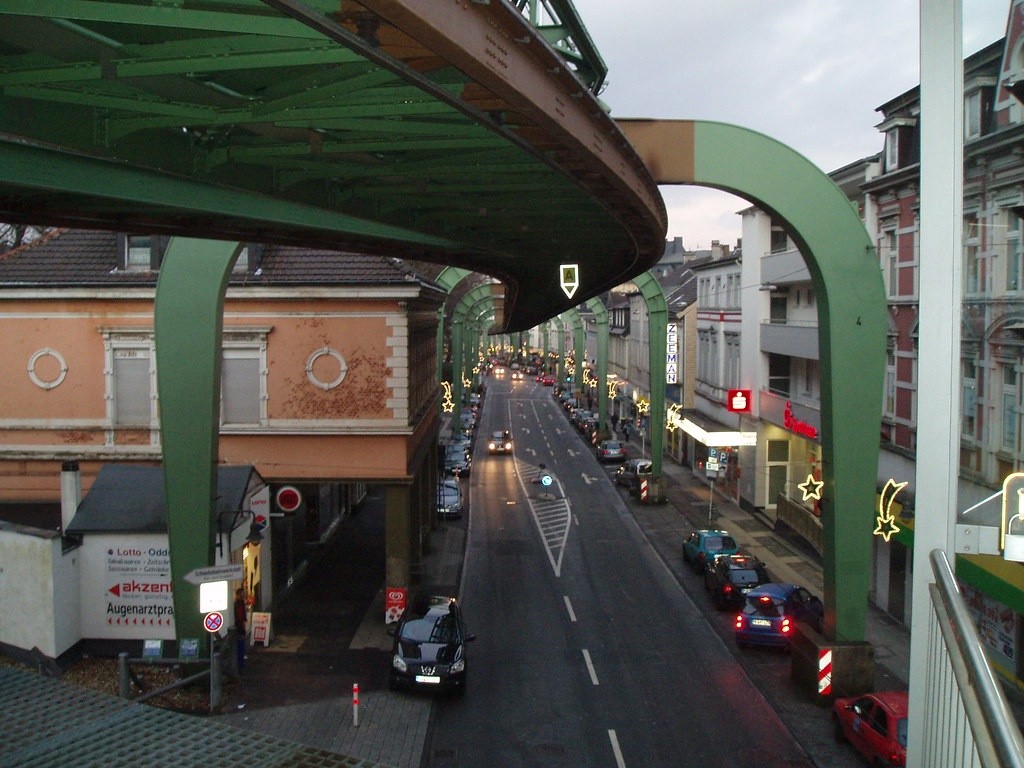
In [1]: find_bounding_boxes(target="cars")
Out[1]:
[703,553,769,612]
[445,445,471,477]
[614,459,653,490]
[487,430,514,455]
[454,393,481,453]
[832,689,910,768]
[733,582,823,651]
[553,385,608,440]
[435,479,464,520]
[485,355,554,387]
[683,529,739,573]
[595,439,627,463]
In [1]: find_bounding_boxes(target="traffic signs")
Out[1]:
[184,563,244,585]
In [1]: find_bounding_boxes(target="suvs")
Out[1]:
[386,593,477,703]
